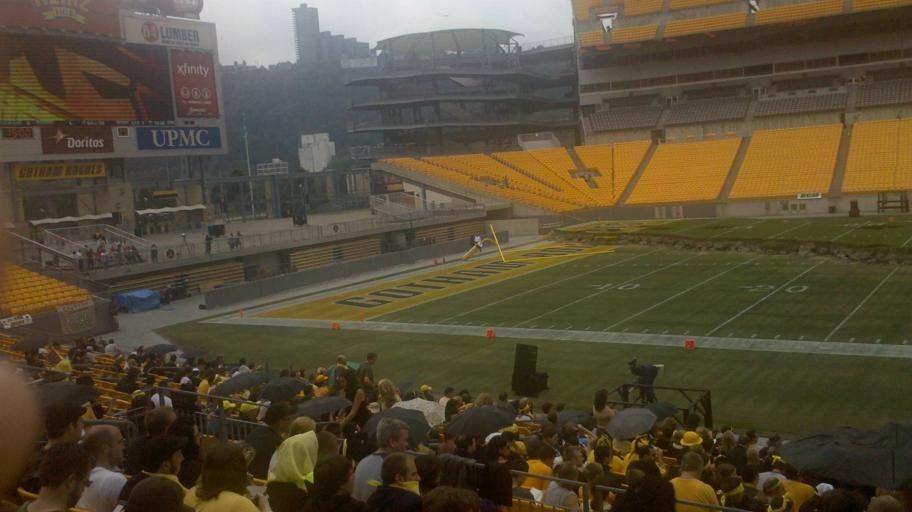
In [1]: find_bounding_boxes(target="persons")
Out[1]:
[228,232,235,250]
[150,244,159,262]
[2,337,911,511]
[235,230,242,248]
[205,234,214,254]
[72,229,144,271]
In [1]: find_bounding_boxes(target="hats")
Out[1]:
[316,374,328,383]
[420,384,432,392]
[679,430,703,447]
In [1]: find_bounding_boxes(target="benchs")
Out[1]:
[0,257,100,323]
